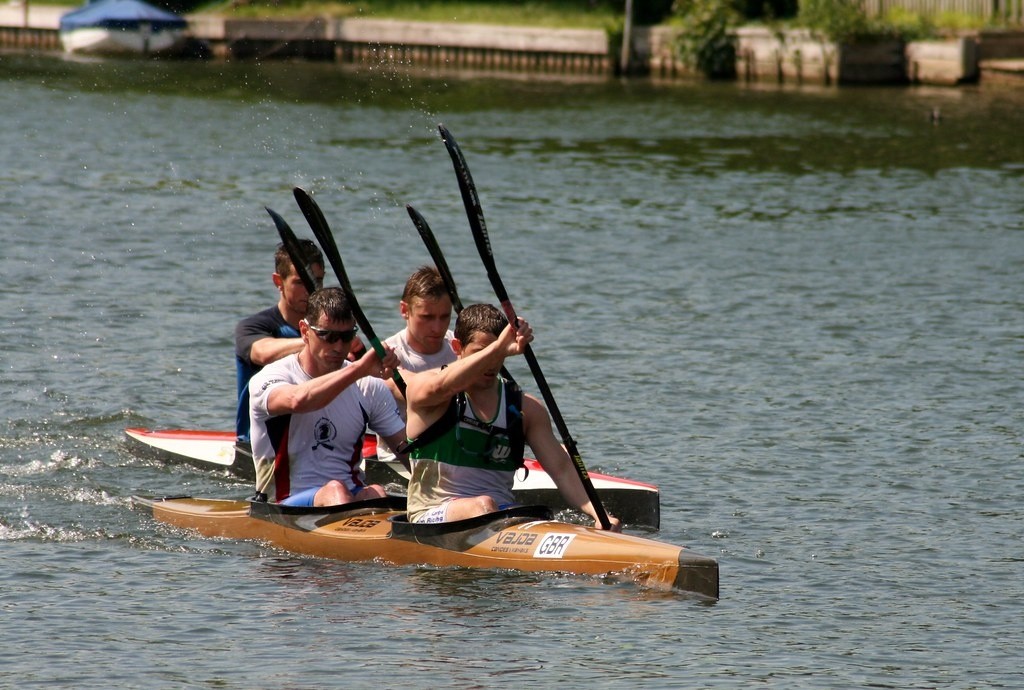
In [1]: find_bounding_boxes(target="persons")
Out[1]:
[406,303,623,534]
[376,266,461,462]
[248,288,408,507]
[237,239,367,444]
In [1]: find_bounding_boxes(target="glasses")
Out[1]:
[302,318,359,344]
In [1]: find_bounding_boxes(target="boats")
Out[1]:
[124,427,660,528]
[132,495,721,600]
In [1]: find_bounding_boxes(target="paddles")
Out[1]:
[264,202,369,363]
[292,182,410,398]
[435,120,613,532]
[405,199,515,386]
